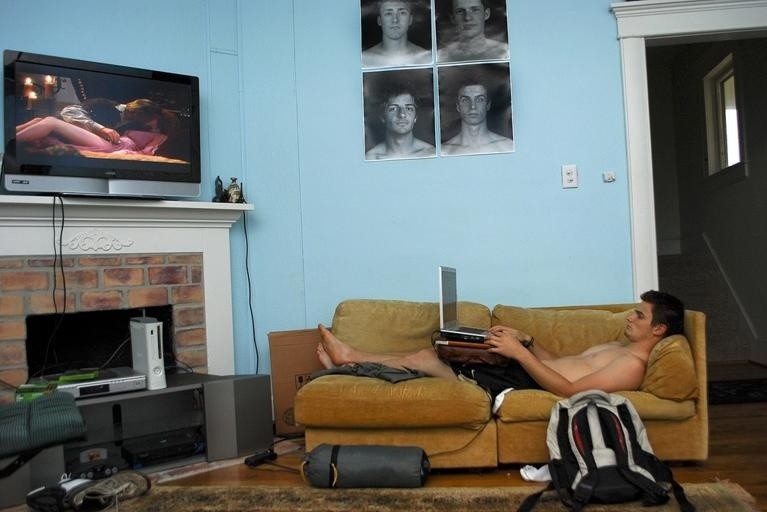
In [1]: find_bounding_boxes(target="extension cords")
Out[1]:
[244,451,277,467]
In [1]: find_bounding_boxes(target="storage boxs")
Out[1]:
[265,328,332,437]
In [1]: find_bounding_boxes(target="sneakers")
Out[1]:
[70,470,151,511]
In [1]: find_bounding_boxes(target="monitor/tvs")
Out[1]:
[3,49,202,199]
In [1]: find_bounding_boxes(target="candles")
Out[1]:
[26,92,37,108]
[23,77,32,97]
[43,75,53,98]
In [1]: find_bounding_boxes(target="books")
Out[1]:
[15,379,57,399]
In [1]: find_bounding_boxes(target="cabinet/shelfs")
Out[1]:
[1,372,271,507]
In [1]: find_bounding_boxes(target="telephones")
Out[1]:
[212,176,227,202]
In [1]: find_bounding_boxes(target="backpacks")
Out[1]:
[515,388,698,512]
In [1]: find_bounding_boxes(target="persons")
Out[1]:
[439,78,514,155]
[61,99,153,144]
[437,0,510,64]
[315,289,684,397]
[16,106,175,155]
[366,81,434,158]
[362,1,432,71]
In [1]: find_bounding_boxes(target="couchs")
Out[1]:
[293,297,713,471]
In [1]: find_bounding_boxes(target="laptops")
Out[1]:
[439,264,494,341]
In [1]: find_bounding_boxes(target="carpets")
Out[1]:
[105,478,764,510]
